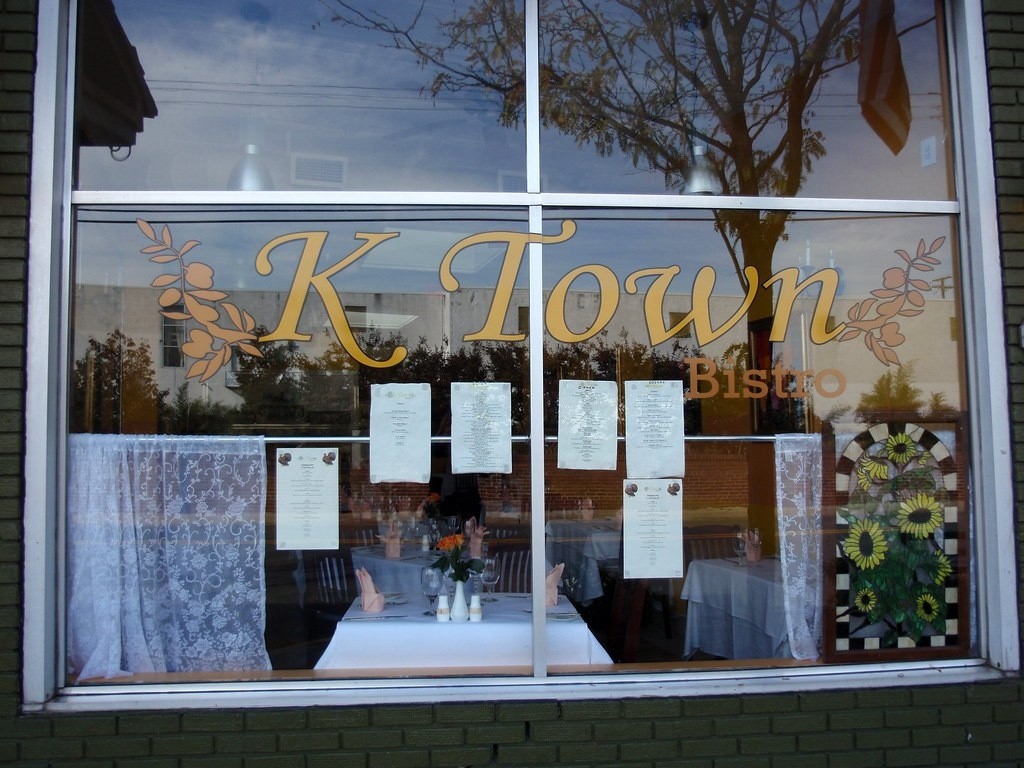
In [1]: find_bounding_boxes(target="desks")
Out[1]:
[680,554,817,659]
[544,519,622,608]
[296,543,488,602]
[312,592,614,665]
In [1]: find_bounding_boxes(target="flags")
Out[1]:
[858,0,912,155]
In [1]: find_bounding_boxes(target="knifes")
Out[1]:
[345,616,408,621]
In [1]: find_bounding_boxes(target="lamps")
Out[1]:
[225,141,273,191]
[683,145,723,195]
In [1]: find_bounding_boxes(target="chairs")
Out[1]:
[487,522,587,593]
[266,520,380,670]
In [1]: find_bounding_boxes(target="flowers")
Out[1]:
[426,493,441,516]
[430,533,485,583]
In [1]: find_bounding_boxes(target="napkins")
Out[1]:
[466,516,478,537]
[356,567,384,612]
[614,509,623,529]
[743,530,761,562]
[386,525,402,559]
[545,562,566,606]
[469,525,490,557]
[582,499,596,521]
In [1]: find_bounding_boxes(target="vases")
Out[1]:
[450,581,469,622]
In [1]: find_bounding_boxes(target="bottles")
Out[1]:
[470,595,482,622]
[422,535,429,551]
[437,596,450,622]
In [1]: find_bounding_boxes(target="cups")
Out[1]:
[745,528,760,562]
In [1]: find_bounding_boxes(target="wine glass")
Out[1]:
[392,516,474,555]
[732,533,747,567]
[479,558,501,602]
[420,567,444,616]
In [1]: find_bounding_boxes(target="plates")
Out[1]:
[546,613,579,621]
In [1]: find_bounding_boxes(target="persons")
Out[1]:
[422,407,481,530]
